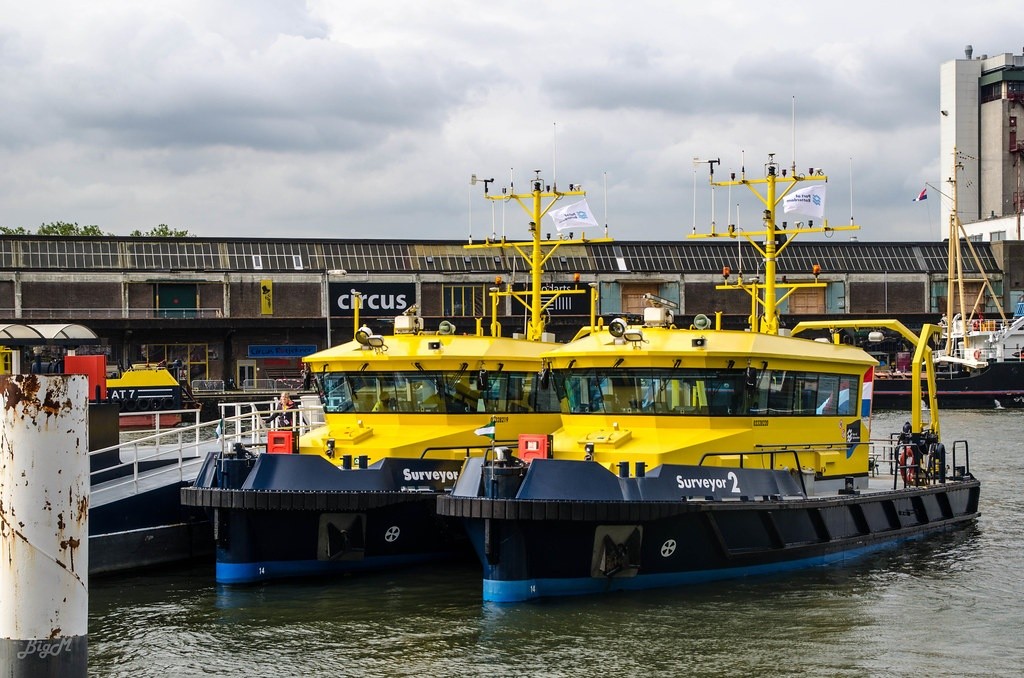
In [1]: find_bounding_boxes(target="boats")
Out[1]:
[699,145,1024,408]
[176,90,981,603]
[1,324,200,427]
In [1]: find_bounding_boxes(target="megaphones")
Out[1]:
[439,321,456,335]
[694,314,711,330]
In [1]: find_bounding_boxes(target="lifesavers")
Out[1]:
[124,398,138,412]
[137,398,150,411]
[974,350,980,360]
[150,397,162,410]
[898,448,915,483]
[163,397,176,410]
[113,399,125,411]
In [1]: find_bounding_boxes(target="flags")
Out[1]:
[215,418,227,439]
[817,366,872,417]
[782,185,824,217]
[913,189,927,201]
[549,199,598,232]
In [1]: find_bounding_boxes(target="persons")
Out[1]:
[262,392,310,434]
[371,392,393,412]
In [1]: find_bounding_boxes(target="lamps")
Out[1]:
[609,318,627,345]
[356,327,373,350]
[623,329,649,350]
[366,335,388,355]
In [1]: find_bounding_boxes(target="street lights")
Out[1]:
[325,269,348,347]
